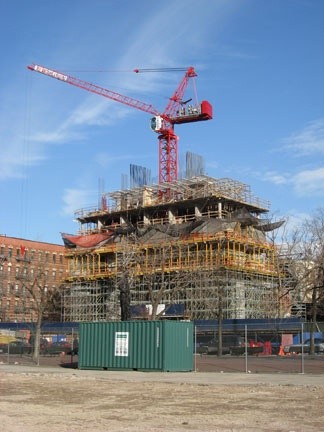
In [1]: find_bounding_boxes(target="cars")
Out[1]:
[0,341,34,354]
[283,338,324,354]
[43,340,73,355]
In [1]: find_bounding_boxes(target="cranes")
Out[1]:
[27,63,214,202]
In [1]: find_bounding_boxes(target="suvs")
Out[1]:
[199,335,246,354]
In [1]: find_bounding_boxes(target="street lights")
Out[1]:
[52,286,63,323]
